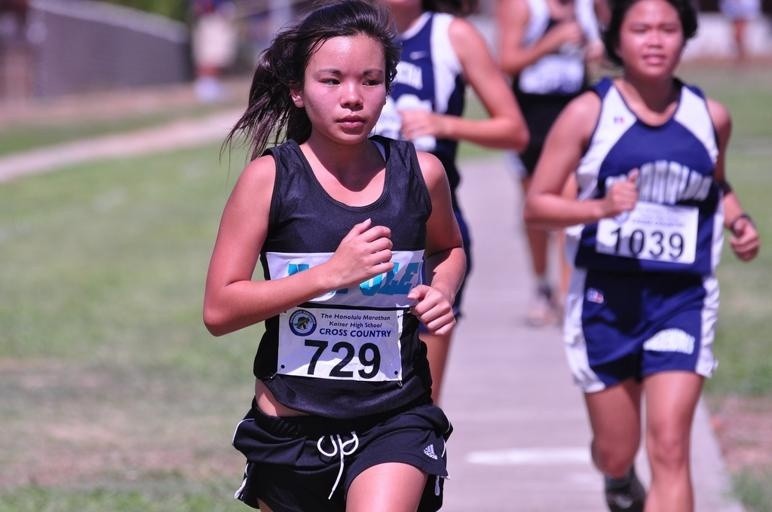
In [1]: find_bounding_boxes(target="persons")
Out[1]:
[494,0,609,333]
[186,1,241,103]
[354,0,532,407]
[199,0,471,512]
[521,0,763,512]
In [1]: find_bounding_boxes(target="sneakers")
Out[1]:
[528,282,568,325]
[603,466,647,512]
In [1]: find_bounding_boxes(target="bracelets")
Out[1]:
[727,211,753,231]
